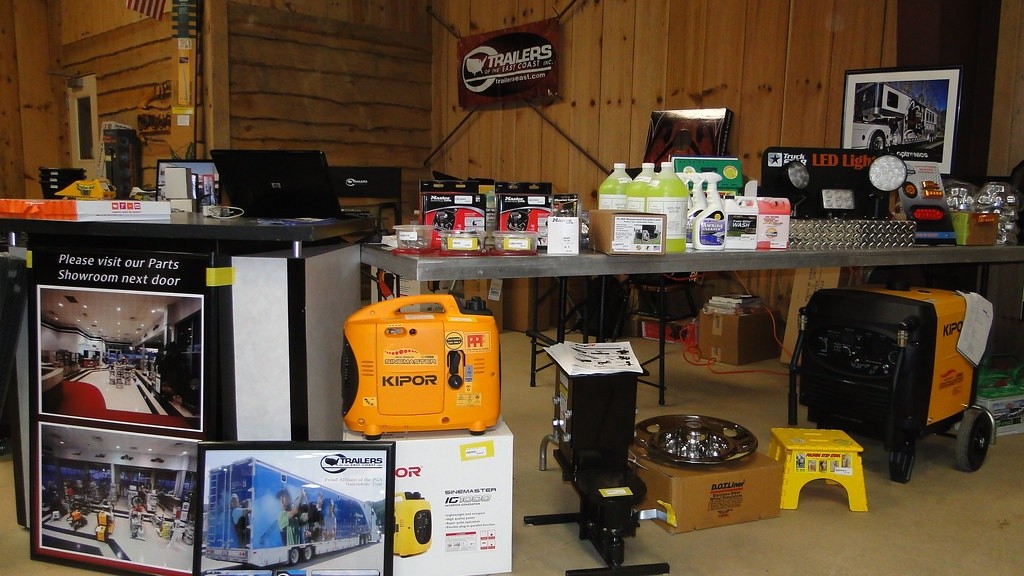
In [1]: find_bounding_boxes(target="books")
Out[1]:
[703,291,763,315]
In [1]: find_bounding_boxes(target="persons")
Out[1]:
[276,486,339,548]
[230,487,255,548]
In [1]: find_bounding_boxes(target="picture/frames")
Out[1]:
[192,440,396,576]
[841,64,964,177]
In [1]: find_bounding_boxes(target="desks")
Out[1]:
[361,244,1024,280]
[0,212,381,531]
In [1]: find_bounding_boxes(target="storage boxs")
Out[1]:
[39,166,87,200]
[698,310,780,365]
[954,375,1024,436]
[341,414,513,576]
[627,444,784,534]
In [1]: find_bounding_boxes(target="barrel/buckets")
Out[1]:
[690,193,759,250]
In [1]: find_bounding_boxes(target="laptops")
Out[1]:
[209,148,370,218]
[760,146,890,221]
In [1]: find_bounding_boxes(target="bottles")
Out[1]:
[626,162,657,212]
[647,162,688,251]
[598,164,632,210]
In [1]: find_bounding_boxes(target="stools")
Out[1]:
[766,428,869,512]
